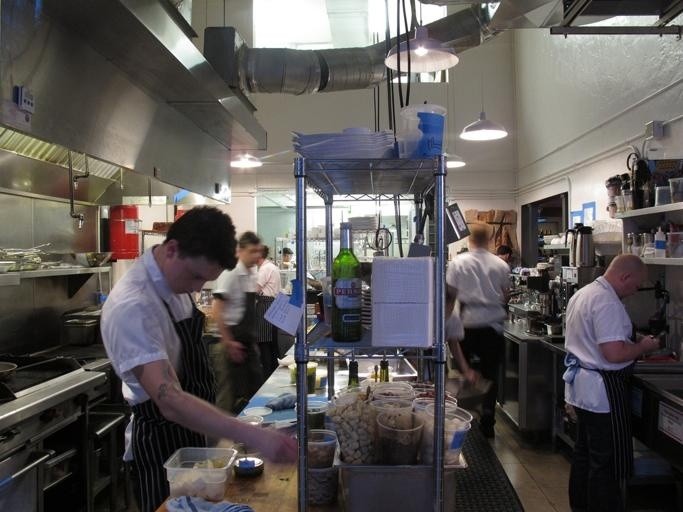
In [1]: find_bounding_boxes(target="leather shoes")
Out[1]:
[478,423,495,439]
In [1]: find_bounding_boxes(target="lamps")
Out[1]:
[382,2,460,76]
[457,2,509,143]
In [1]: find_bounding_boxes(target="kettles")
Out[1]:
[564,223,595,266]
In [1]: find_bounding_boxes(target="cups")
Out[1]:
[615,195,632,213]
[294,383,474,512]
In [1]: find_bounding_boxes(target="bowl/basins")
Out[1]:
[0,362,18,378]
[70,252,114,267]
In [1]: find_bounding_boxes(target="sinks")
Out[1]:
[663,386,683,405]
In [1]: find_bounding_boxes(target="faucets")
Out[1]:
[71,151,89,190]
[66,149,85,232]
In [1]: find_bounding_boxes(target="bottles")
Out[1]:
[627,227,666,257]
[348,359,388,388]
[329,222,363,343]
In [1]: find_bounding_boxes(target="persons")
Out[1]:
[288,263,315,294]
[208,231,261,417]
[496,245,514,274]
[404,283,476,385]
[254,245,282,379]
[277,247,294,289]
[562,254,661,512]
[98,204,299,512]
[445,223,510,441]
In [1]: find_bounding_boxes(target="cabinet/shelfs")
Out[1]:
[289,154,449,510]
